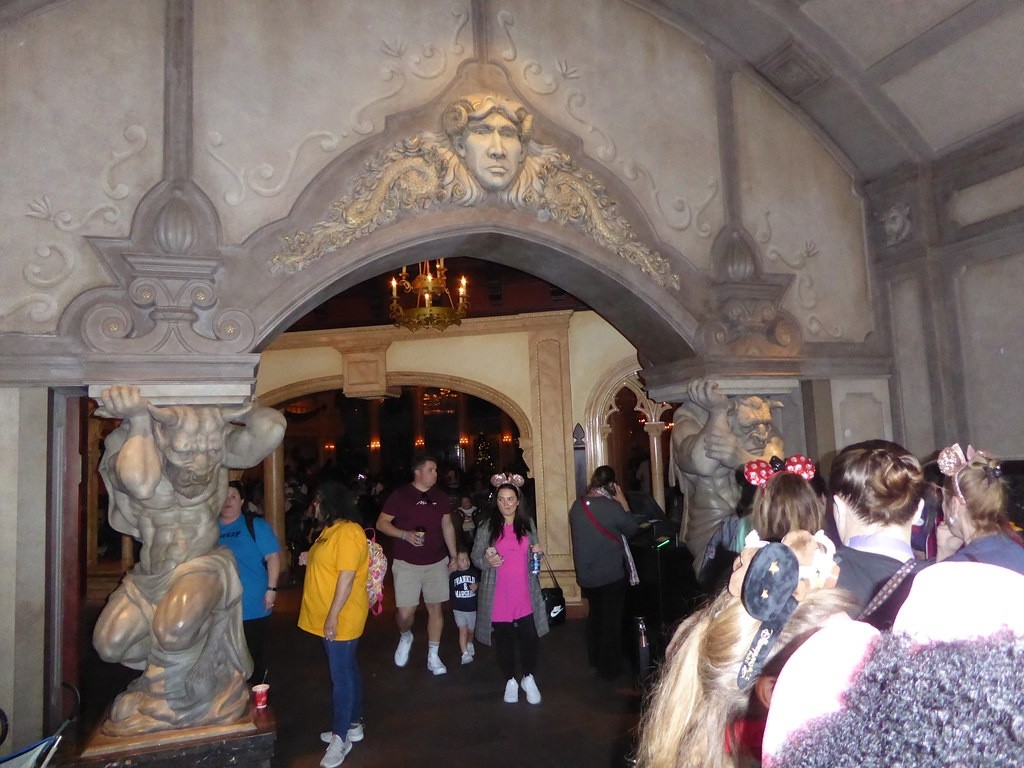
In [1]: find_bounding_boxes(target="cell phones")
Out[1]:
[610,482,616,495]
[486,547,497,556]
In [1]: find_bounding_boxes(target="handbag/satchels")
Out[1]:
[622,534,640,586]
[541,588,566,626]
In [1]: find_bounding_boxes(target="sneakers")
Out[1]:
[320,734,352,768]
[520,673,542,705]
[320,723,364,743]
[396,632,414,666]
[504,677,519,703]
[428,656,447,675]
[467,643,476,655]
[461,651,473,664]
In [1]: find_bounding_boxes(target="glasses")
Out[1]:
[421,492,433,505]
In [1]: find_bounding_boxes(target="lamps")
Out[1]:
[389,260,468,335]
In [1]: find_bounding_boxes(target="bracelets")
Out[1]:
[401,531,407,540]
[267,587,277,591]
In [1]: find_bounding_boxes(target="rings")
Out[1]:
[272,604,274,607]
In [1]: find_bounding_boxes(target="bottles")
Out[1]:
[530,547,541,575]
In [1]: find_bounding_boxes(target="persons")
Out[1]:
[472,475,544,705]
[299,478,369,768]
[443,94,533,191]
[242,451,482,675]
[640,438,1024,767]
[215,481,280,659]
[568,466,637,680]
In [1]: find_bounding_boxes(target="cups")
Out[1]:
[252,684,270,708]
[415,526,425,545]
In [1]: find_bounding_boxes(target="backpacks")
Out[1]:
[364,528,388,617]
